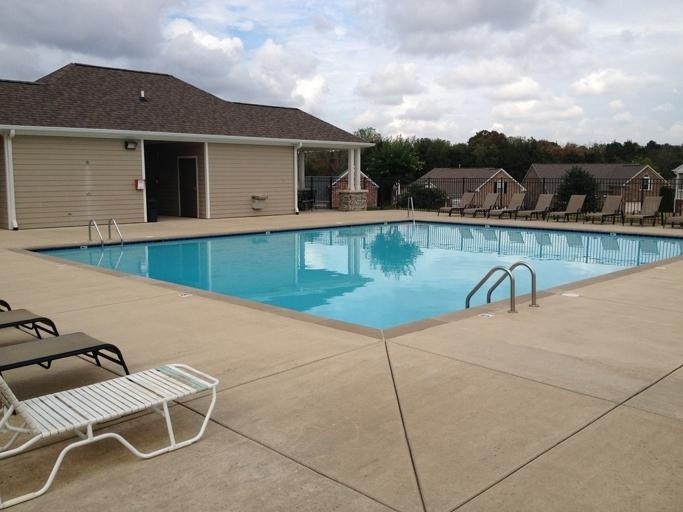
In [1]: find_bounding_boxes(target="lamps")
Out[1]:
[124,141,137,150]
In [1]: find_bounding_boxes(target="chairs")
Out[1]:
[437,192,683,229]
